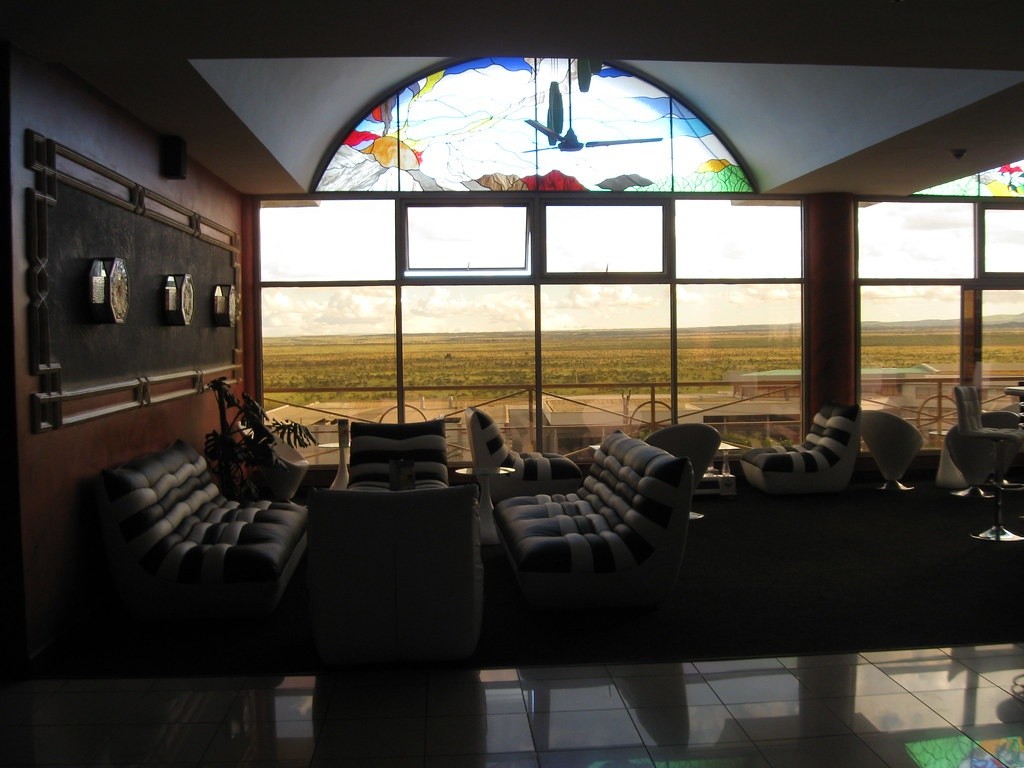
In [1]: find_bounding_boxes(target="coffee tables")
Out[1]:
[692,433,741,497]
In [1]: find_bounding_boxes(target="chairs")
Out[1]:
[943,410,1021,498]
[644,423,723,520]
[861,409,924,492]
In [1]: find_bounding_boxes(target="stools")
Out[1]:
[953,384,1024,542]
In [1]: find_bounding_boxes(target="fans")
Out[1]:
[522,60,664,155]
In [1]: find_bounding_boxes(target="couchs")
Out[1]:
[491,429,695,608]
[76,438,309,646]
[306,484,488,667]
[465,406,584,500]
[741,402,863,496]
[342,420,450,496]
